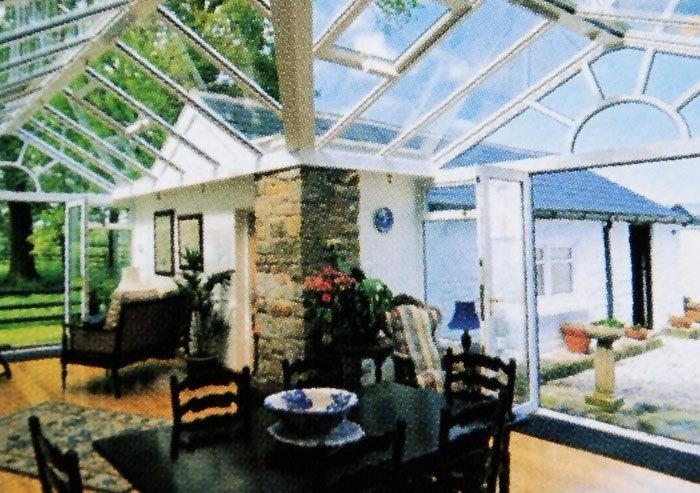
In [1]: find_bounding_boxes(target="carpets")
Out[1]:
[0,399,176,490]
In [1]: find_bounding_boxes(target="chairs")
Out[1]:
[384,294,455,391]
[29,345,518,491]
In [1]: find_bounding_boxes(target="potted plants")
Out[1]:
[174,247,237,376]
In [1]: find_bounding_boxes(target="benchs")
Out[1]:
[60,278,194,400]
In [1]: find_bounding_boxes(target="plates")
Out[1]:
[267,420,368,447]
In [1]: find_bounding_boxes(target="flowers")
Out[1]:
[299,267,357,304]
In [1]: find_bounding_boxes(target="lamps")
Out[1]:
[446,298,480,349]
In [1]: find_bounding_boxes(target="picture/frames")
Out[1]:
[178,211,204,271]
[153,207,175,278]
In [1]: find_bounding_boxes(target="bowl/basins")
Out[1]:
[263,385,361,436]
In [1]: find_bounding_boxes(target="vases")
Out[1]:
[315,307,333,343]
[562,323,590,351]
